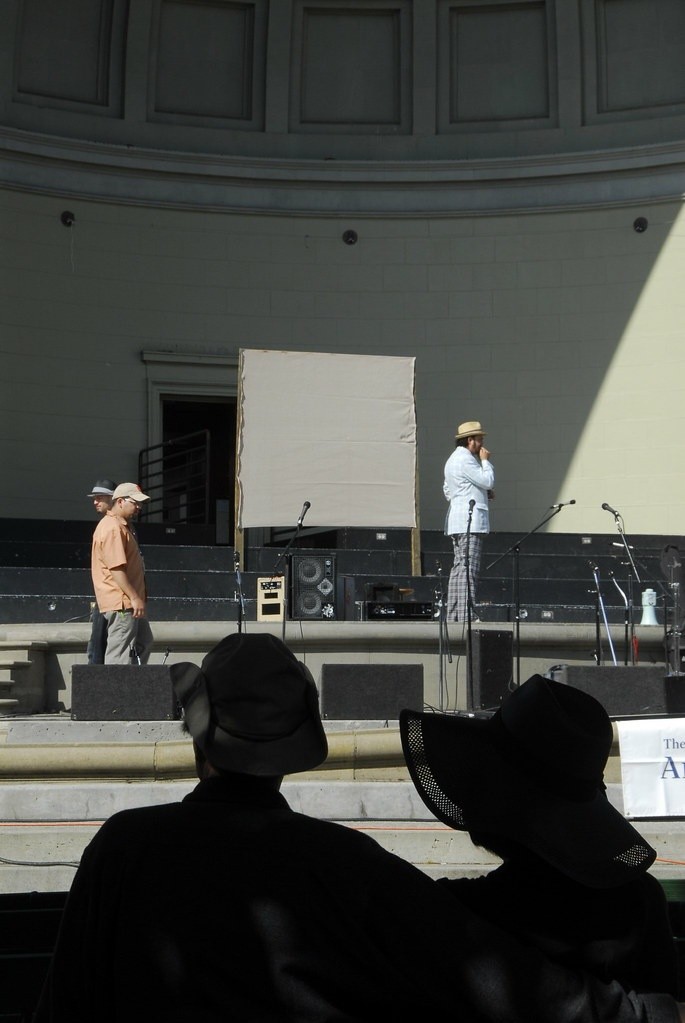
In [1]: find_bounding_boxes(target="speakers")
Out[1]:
[466,630,515,711]
[291,555,337,621]
[320,663,426,719]
[71,663,182,721]
[548,665,668,715]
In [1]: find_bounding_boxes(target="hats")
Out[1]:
[86,479,117,497]
[169,631,328,777]
[399,672,655,887]
[455,421,487,439]
[111,483,152,503]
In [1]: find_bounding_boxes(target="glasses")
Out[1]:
[124,497,144,506]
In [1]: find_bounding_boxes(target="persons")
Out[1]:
[443,421,495,622]
[31,633,685,1023]
[86,478,117,664]
[399,673,680,998]
[92,482,153,664]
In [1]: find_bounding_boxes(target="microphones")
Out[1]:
[234,550,240,565]
[585,557,599,572]
[550,500,576,510]
[298,501,311,527]
[469,499,476,516]
[602,503,620,516]
